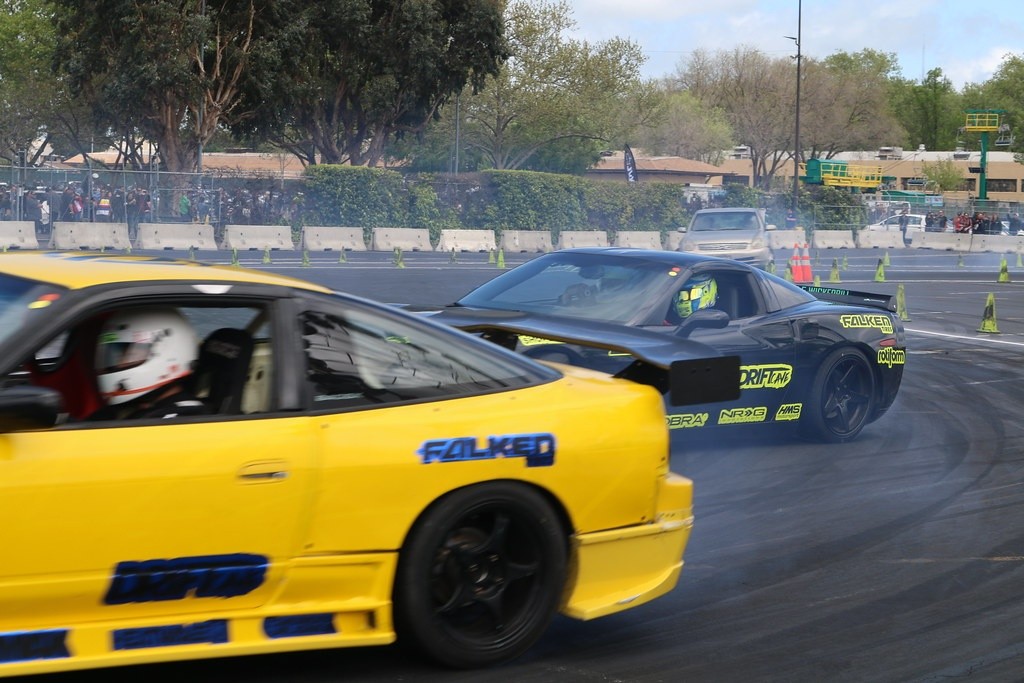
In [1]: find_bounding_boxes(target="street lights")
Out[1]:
[784,35,803,226]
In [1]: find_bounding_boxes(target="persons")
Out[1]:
[93,301,211,419]
[866,202,908,225]
[1007,209,1020,235]
[785,208,797,229]
[690,191,702,214]
[663,272,719,326]
[925,210,948,232]
[0,182,305,224]
[954,211,1003,234]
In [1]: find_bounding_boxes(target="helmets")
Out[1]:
[94,305,199,407]
[671,269,718,318]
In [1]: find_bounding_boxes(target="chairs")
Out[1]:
[714,282,739,320]
[735,218,756,231]
[699,216,716,231]
[183,327,256,416]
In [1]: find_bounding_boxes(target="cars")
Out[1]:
[985,220,1024,236]
[864,214,957,233]
[673,207,778,273]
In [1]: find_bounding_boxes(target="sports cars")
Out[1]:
[363,246,910,442]
[0,247,694,683]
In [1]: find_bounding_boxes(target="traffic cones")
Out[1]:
[872,257,885,282]
[813,273,822,288]
[882,251,891,266]
[262,248,273,264]
[392,247,405,269]
[186,247,196,260]
[300,247,311,267]
[340,247,350,264]
[841,252,850,269]
[488,247,507,269]
[975,292,1000,333]
[958,252,965,268]
[997,257,1010,283]
[893,284,912,322]
[828,257,842,285]
[231,247,240,266]
[1016,252,1023,268]
[791,244,814,284]
[784,262,794,282]
